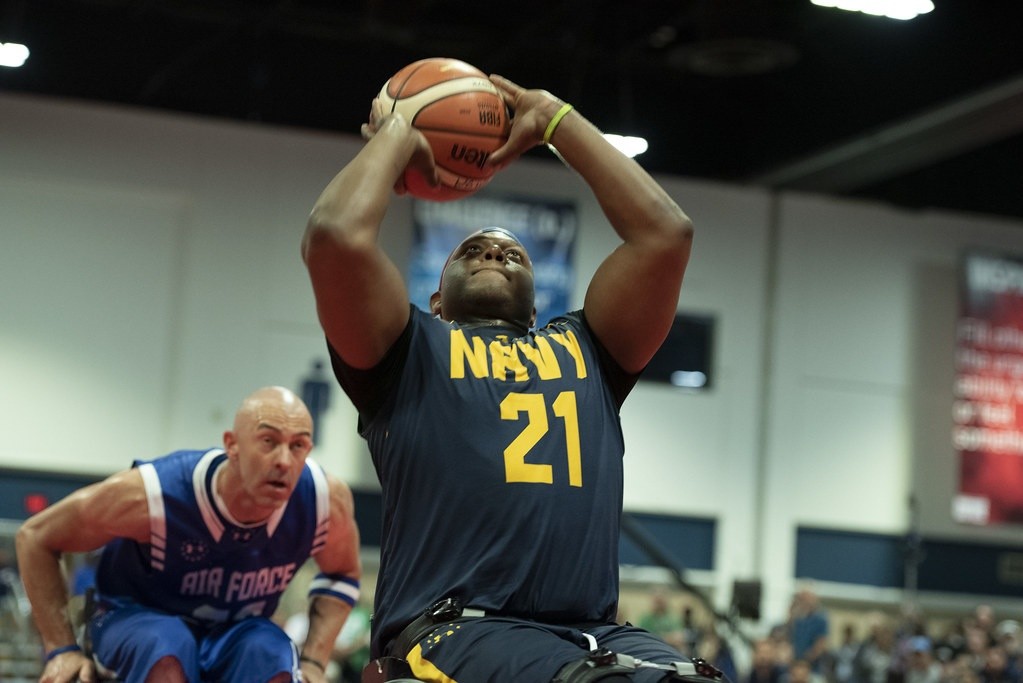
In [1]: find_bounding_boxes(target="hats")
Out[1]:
[991,620,1019,641]
[793,590,816,608]
[900,635,930,658]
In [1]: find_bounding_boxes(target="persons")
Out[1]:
[17,385,362,683]
[643,587,1023,683]
[300,73,727,683]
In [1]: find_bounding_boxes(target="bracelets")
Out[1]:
[543,103,573,146]
[299,657,326,674]
[44,644,80,665]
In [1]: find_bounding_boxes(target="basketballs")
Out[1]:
[372,57,510,203]
